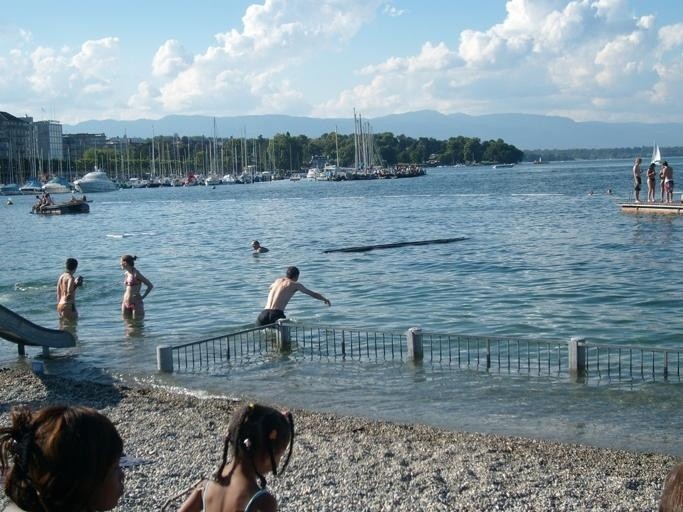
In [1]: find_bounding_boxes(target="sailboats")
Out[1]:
[0,116,290,195]
[290,107,426,181]
[652,140,661,164]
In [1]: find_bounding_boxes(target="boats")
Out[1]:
[493,164,514,169]
[616,200,683,214]
[34,197,89,214]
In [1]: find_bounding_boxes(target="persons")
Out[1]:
[125,321,143,349]
[251,240,268,253]
[0,404,126,512]
[257,267,331,330]
[56,259,81,333]
[587,158,674,203]
[178,403,294,512]
[659,466,683,512]
[7,192,86,214]
[121,255,153,321]
